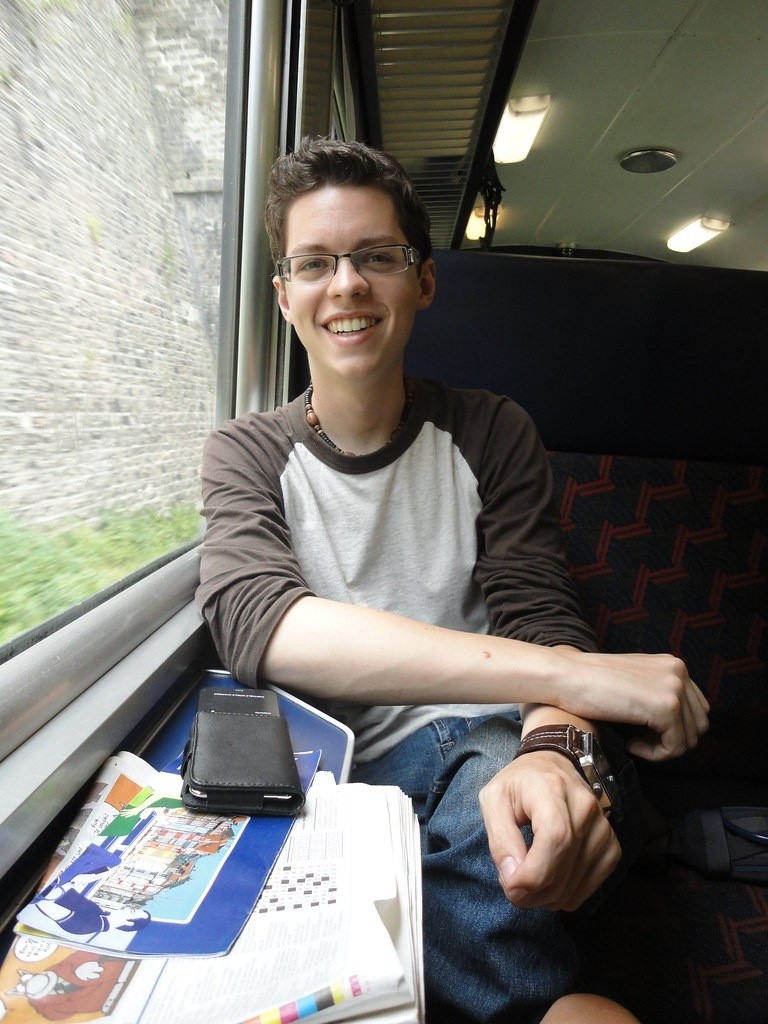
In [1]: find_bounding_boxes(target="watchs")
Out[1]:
[512,721,620,819]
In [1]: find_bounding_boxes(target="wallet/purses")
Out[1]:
[179,711,306,817]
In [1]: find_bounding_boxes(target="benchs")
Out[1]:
[405,249,768,1024]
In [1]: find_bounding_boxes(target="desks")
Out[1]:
[0,663,357,1024]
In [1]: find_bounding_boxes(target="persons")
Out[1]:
[194,136,711,1024]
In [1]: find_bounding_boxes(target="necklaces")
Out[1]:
[304,373,414,459]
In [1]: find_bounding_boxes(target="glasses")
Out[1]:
[275,243,423,284]
